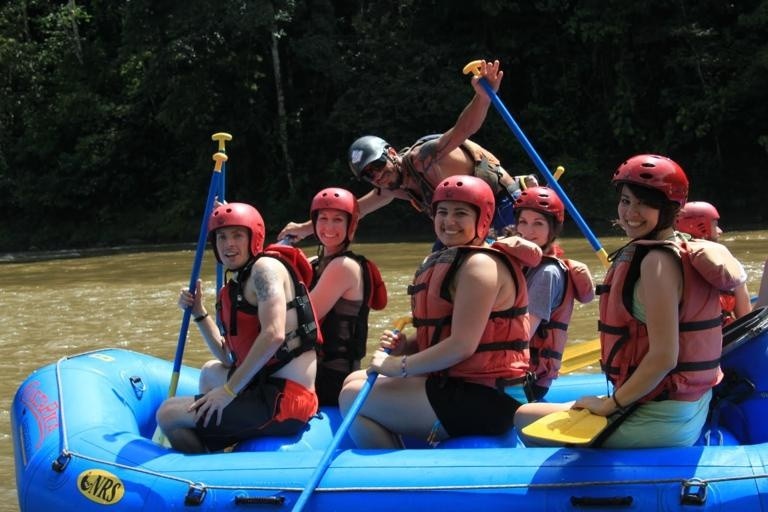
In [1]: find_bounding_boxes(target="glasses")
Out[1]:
[362,153,386,181]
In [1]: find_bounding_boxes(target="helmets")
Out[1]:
[611,154,689,208]
[348,135,392,183]
[674,201,720,239]
[513,186,565,224]
[310,188,360,241]
[432,175,495,240]
[208,202,265,264]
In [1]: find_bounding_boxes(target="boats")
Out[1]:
[6,328,767,512]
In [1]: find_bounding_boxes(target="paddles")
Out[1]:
[557,297,760,374]
[523,392,609,445]
[151,153,229,448]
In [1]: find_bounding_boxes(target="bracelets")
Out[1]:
[612,391,625,411]
[401,355,407,379]
[223,384,237,399]
[193,313,208,323]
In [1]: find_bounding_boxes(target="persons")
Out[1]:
[338,175,544,448]
[754,259,768,309]
[156,203,325,454]
[513,153,748,447]
[673,202,753,329]
[512,185,596,403]
[277,59,523,244]
[305,187,387,407]
